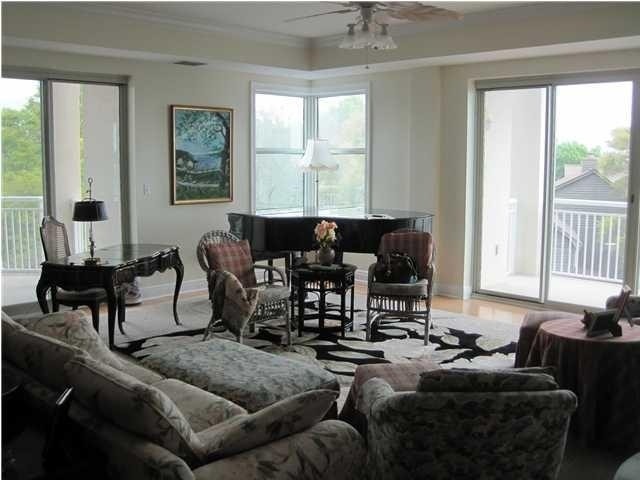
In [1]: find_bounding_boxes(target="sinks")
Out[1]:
[3,308,364,480]
[605,288,640,324]
[355,363,581,476]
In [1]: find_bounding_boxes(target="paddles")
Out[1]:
[66,287,527,417]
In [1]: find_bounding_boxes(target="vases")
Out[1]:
[315,220,342,244]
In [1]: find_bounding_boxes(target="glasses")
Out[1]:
[72,178,114,267]
[338,15,395,70]
[300,136,336,214]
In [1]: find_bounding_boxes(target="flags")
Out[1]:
[317,247,337,266]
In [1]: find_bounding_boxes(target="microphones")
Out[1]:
[337,362,442,424]
[512,311,582,369]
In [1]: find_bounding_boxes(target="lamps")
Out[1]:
[365,230,436,341]
[36,214,126,339]
[195,227,292,351]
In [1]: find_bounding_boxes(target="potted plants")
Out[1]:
[169,104,236,208]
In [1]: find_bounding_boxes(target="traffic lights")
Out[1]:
[203,240,260,290]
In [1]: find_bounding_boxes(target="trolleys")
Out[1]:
[281,3,466,28]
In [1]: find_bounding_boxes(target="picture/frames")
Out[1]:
[36,243,186,342]
[228,206,436,325]
[296,262,358,338]
[518,317,640,451]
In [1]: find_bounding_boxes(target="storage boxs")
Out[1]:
[140,335,338,420]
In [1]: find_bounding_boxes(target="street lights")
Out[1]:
[375,252,418,283]
